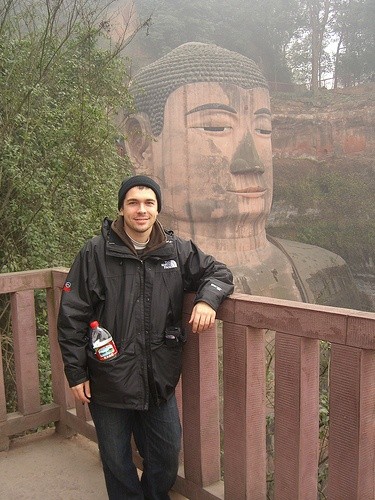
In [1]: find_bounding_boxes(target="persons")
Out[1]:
[55,174,233,500]
[119,41,361,315]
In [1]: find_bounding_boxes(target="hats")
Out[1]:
[118,174,162,214]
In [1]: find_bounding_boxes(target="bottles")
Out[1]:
[87,319,119,362]
